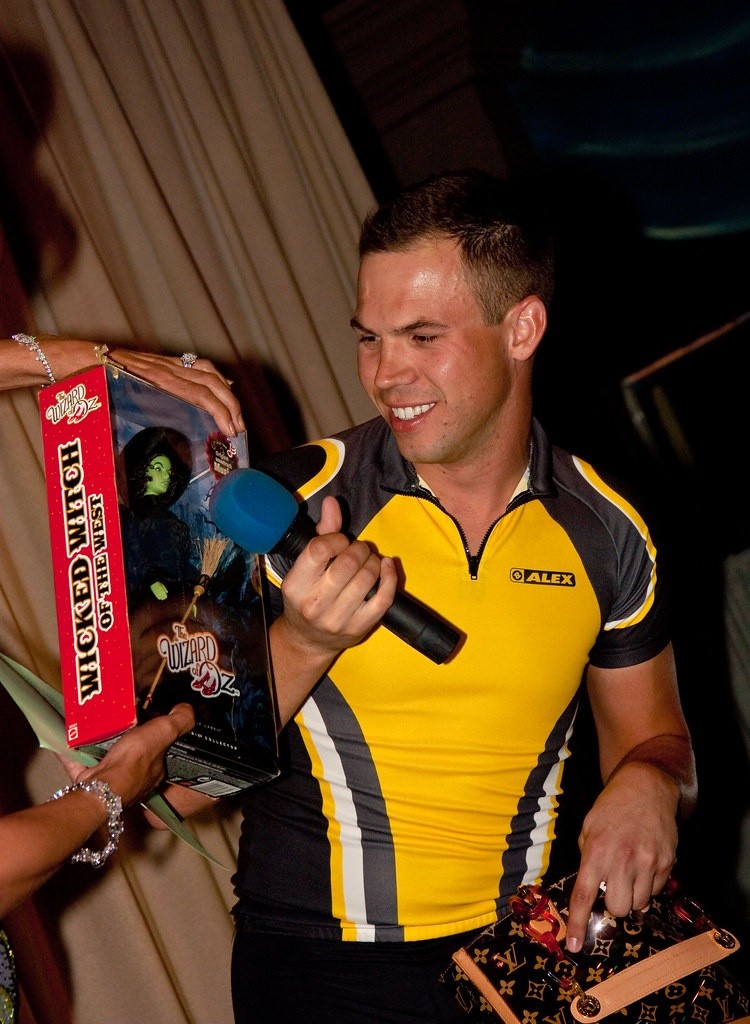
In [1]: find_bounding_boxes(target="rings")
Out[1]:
[181,351,198,368]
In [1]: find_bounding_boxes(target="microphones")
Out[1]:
[209,466,461,666]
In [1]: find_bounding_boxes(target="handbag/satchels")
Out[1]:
[438,870,750,1024]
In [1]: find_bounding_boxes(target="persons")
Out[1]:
[114,427,199,613]
[1,329,244,1024]
[144,160,698,1024]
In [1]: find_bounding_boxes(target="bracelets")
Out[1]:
[12,332,58,388]
[47,776,126,870]
